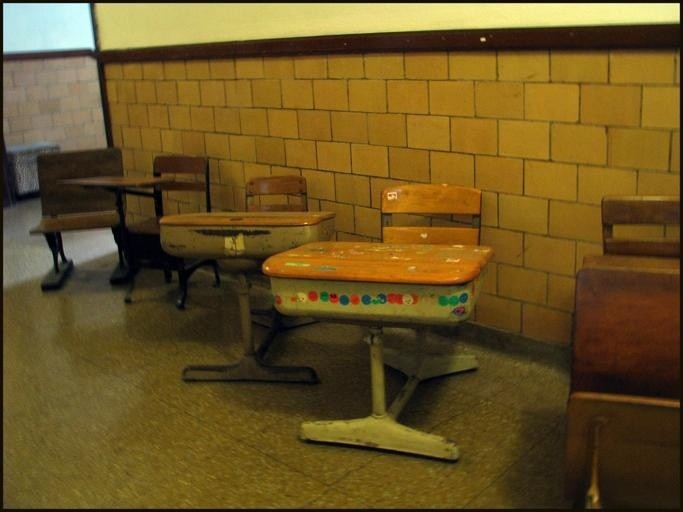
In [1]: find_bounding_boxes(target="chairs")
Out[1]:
[118,153,220,310]
[244,176,316,323]
[564,193,683,512]
[32,147,124,290]
[365,184,482,380]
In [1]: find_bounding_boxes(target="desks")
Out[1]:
[5,143,61,196]
[61,171,173,286]
[158,210,336,383]
[262,242,496,459]
